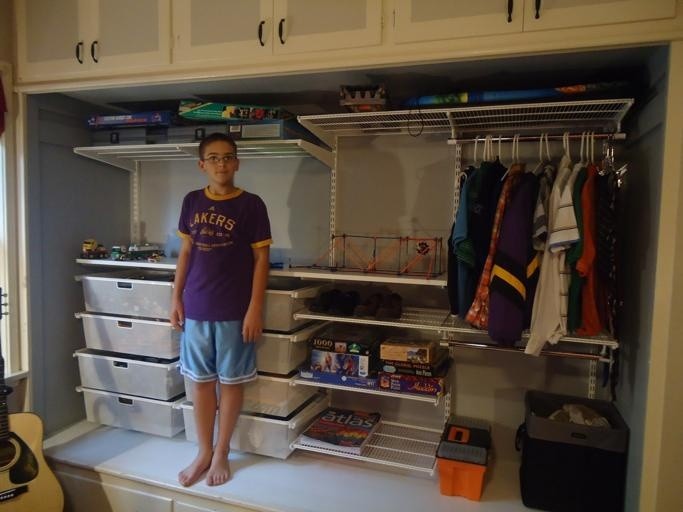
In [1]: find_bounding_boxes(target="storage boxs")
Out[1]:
[435,412,491,501]
[244,320,328,376]
[514,388,630,511]
[92,119,321,146]
[73,312,184,359]
[73,347,185,400]
[179,370,319,418]
[179,395,328,462]
[253,281,328,332]
[75,384,185,440]
[74,274,177,320]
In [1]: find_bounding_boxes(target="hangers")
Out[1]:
[457,133,602,185]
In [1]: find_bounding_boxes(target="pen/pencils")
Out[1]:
[296,265,313,268]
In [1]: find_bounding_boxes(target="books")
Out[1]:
[299,407,382,455]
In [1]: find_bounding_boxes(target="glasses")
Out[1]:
[203,155,236,164]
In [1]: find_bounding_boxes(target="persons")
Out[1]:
[169,133,273,489]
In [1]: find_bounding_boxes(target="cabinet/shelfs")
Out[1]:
[289,305,457,476]
[8,0,683,113]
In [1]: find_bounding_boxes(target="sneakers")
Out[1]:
[309,288,402,321]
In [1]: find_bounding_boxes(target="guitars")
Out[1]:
[1,287,66,512]
